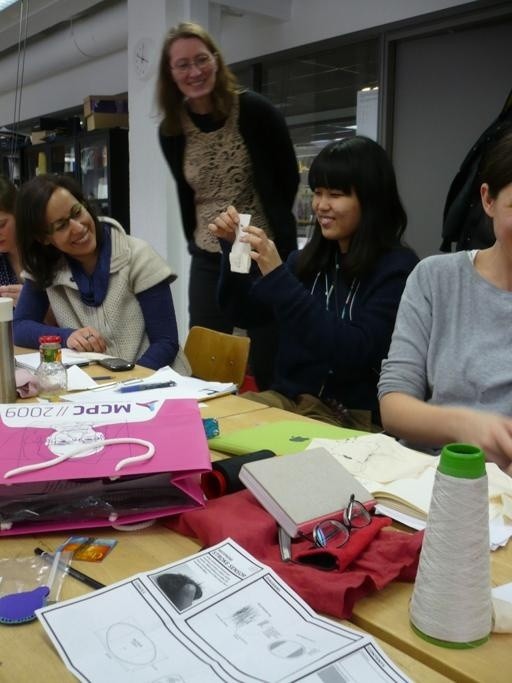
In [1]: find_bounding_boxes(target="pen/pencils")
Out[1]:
[92,376,115,380]
[66,361,98,369]
[34,547,106,590]
[118,381,176,393]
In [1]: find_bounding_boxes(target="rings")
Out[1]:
[85,333,94,340]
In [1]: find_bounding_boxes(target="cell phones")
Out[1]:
[97,356,135,373]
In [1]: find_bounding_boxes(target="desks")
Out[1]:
[0,338,512,681]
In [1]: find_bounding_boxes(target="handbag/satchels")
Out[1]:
[0,401,213,537]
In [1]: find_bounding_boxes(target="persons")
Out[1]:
[10,173,198,381]
[377,127,511,479]
[0,176,24,296]
[152,21,304,338]
[207,132,420,435]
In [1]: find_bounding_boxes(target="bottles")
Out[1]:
[0,296,19,403]
[32,334,68,398]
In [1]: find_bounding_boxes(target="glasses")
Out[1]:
[51,202,84,232]
[298,495,371,549]
[170,54,213,71]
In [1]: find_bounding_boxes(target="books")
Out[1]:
[309,429,441,531]
[14,347,119,369]
[236,446,374,539]
[59,362,239,404]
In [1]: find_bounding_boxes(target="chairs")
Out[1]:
[183,326,251,395]
